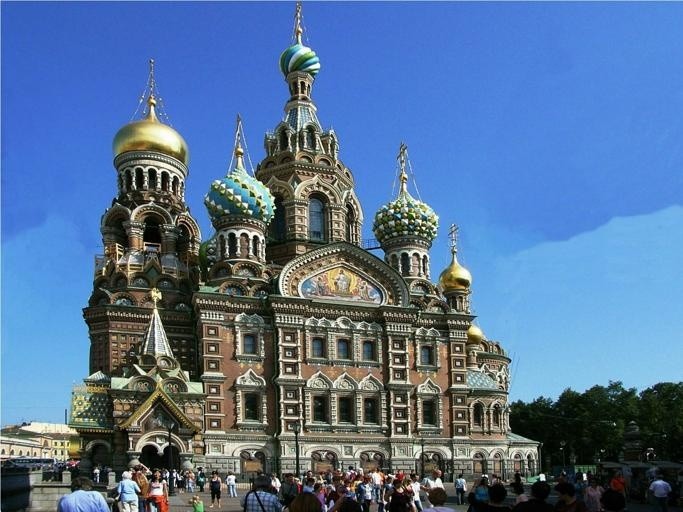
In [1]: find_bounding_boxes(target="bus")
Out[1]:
[9,456,55,470]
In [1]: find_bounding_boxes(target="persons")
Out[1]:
[315,270,372,300]
[231,287,237,296]
[33,459,238,511]
[240,466,682,511]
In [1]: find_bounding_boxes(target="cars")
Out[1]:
[66,459,79,466]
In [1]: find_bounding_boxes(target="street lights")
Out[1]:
[538,441,544,474]
[166,420,176,496]
[419,436,426,479]
[291,419,302,478]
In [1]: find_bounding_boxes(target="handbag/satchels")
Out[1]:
[463,483,468,492]
